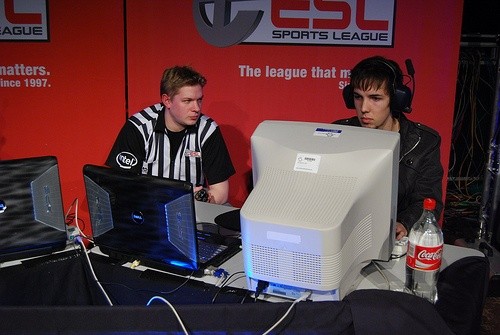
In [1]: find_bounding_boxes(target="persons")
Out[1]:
[104,66,236,205]
[331,56,445,241]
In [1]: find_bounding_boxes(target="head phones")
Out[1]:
[343,58,412,113]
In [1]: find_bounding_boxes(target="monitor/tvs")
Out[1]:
[238,120,400,302]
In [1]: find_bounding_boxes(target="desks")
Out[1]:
[87,198,489,335]
[0,242,86,308]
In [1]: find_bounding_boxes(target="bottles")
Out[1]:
[406,199,443,302]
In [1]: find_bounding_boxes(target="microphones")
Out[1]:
[405,58,415,96]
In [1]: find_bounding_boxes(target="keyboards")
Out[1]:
[391,237,409,258]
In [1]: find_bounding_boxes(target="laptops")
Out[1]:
[83,164,242,277]
[0,156,67,263]
